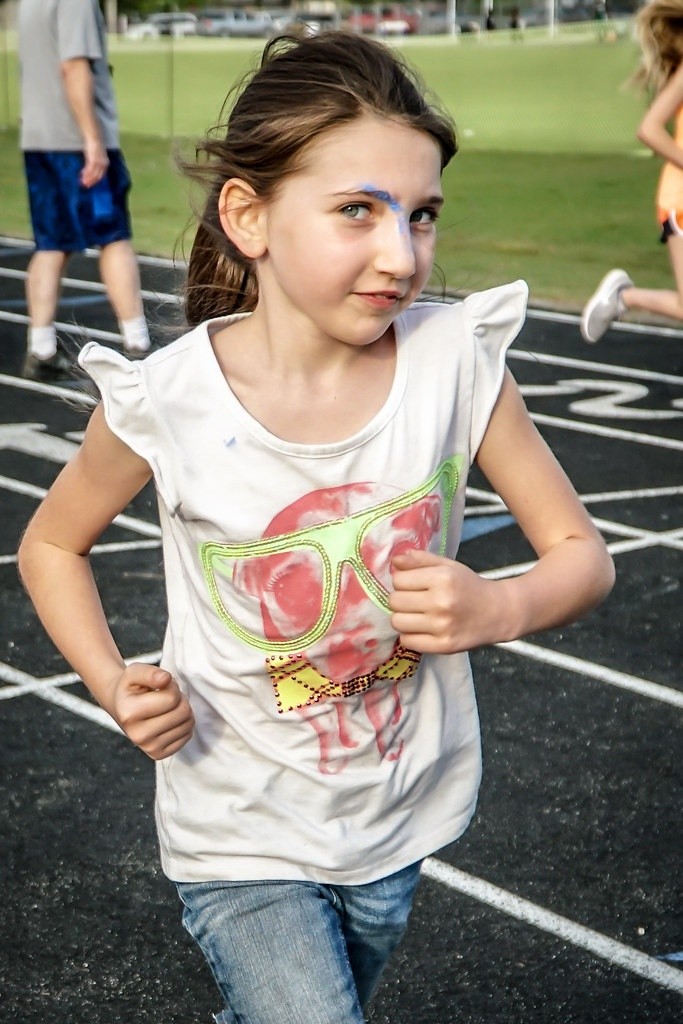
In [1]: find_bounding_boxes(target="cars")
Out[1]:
[131,0,421,41]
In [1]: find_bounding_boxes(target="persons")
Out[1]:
[18,0,161,381]
[580,0,683,343]
[18,32,615,1024]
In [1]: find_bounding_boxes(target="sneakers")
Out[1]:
[582,269,634,345]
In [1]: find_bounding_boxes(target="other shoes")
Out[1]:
[23,350,84,385]
[122,342,162,364]
